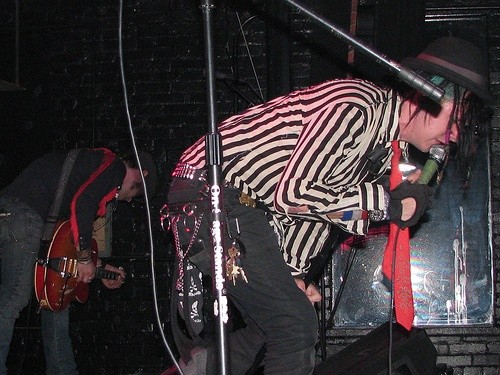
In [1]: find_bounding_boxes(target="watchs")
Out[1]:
[368,210,384,222]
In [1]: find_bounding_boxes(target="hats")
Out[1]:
[401,36,493,103]
[136,148,164,199]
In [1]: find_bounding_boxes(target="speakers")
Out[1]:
[312,321,439,375]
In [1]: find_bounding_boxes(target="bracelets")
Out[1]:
[78,256,92,264]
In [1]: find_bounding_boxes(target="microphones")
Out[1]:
[414,144,447,186]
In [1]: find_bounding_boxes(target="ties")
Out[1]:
[381,139,415,331]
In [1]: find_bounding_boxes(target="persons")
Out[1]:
[167,39,492,375]
[0,149,159,375]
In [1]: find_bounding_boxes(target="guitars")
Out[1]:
[34,219,125,313]
